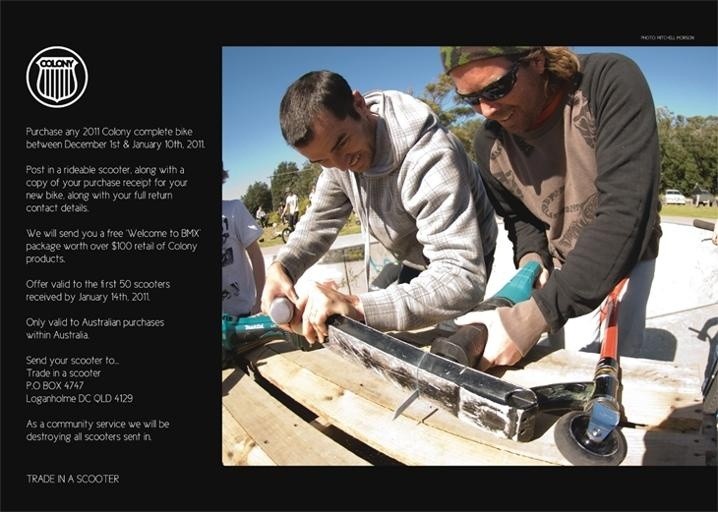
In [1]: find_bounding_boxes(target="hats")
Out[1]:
[440,46,545,76]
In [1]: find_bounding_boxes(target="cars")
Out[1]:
[692,189,715,202]
[665,188,686,205]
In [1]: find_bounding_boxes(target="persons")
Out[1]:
[256,206,268,228]
[309,183,316,207]
[280,187,299,232]
[278,202,289,226]
[260,70,498,346]
[222,162,266,319]
[440,46,663,371]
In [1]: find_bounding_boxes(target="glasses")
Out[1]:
[455,60,523,107]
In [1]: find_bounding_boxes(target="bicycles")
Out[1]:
[281,215,293,244]
